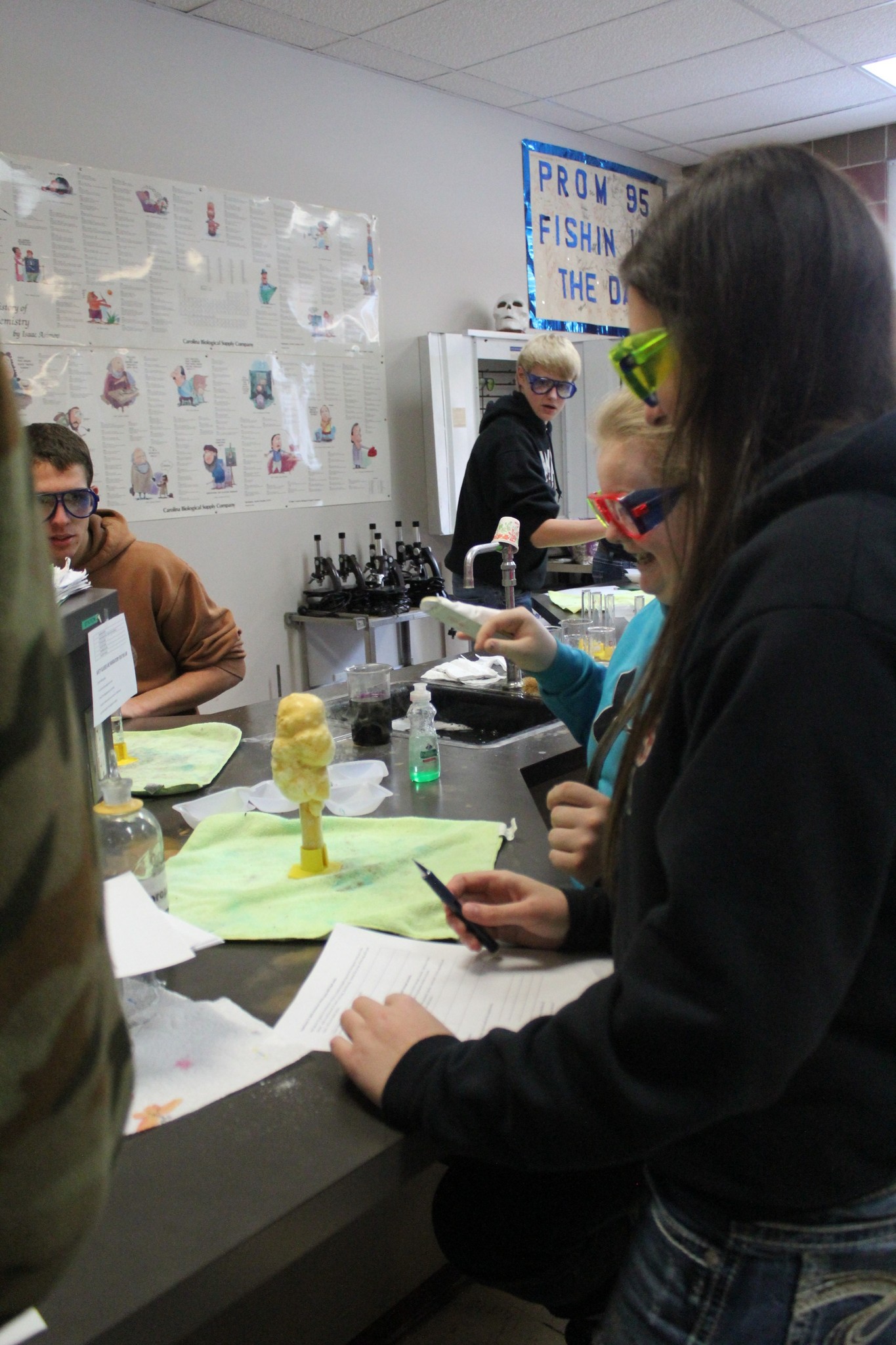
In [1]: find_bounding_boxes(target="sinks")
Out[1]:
[323,676,569,746]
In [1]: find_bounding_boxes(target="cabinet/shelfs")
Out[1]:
[416,328,626,538]
[283,604,447,692]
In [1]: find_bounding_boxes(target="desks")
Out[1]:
[1,651,589,1345]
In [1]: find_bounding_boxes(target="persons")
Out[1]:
[442,331,608,655]
[22,420,250,721]
[462,368,695,894]
[3,347,133,1327]
[324,140,896,1345]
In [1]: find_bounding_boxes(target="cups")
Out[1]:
[347,664,395,745]
[111,713,127,763]
[588,628,615,672]
[545,626,563,643]
[559,618,593,656]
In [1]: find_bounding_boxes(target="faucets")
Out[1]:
[462,535,525,691]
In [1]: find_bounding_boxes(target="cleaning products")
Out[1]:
[405,681,442,783]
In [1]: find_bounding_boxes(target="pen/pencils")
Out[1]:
[411,858,500,954]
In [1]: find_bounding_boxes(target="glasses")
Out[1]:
[34,488,99,523]
[586,481,696,541]
[522,367,577,399]
[610,326,680,404]
